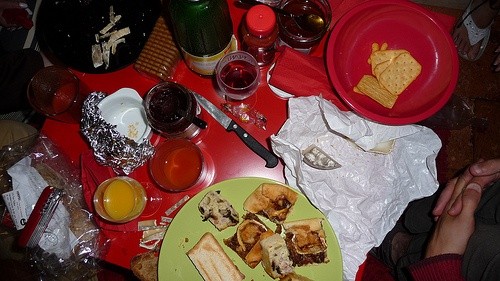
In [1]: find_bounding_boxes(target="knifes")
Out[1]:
[191,90,278,168]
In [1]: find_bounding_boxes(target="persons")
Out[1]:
[0,0,59,237]
[451,0,500,72]
[369,156,500,281]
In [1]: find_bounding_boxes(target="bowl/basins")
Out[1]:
[97,87,151,146]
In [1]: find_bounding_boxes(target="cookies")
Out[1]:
[134,17,180,82]
[354,43,421,109]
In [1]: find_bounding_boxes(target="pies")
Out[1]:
[198,183,331,268]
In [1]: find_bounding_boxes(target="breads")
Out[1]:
[260,233,314,281]
[187,232,245,281]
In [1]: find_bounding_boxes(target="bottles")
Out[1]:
[163,0,242,76]
[239,5,277,66]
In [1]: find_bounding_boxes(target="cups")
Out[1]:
[279,0,332,48]
[144,81,207,136]
[216,50,260,100]
[26,66,88,123]
[150,139,208,192]
[92,177,148,224]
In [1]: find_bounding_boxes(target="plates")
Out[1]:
[326,0,459,125]
[158,177,342,281]
[31,1,162,73]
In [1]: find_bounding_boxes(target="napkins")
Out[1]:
[269,45,354,111]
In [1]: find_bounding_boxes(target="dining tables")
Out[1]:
[0,0,456,281]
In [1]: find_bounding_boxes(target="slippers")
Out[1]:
[452,2,494,60]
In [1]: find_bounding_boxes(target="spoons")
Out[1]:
[238,0,325,35]
